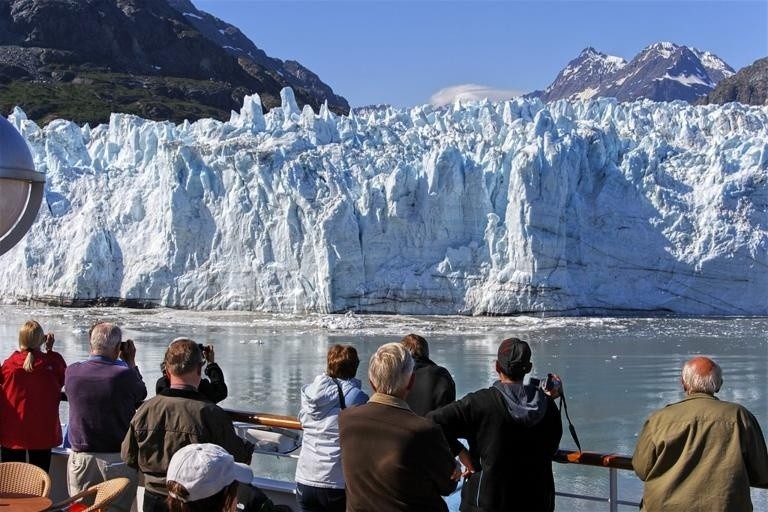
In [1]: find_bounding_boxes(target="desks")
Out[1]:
[0,492,54,512]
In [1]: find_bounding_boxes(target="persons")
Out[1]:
[630,357,768,512]
[399,334,459,497]
[420,339,564,511]
[0,319,67,475]
[339,341,462,511]
[63,322,253,512]
[292,343,371,512]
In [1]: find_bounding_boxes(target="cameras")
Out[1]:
[529,373,561,392]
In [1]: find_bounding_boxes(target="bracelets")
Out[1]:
[48,349,51,351]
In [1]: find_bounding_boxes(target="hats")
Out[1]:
[498,338,532,373]
[166,442,253,502]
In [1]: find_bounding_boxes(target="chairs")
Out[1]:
[0,462,51,498]
[42,477,131,512]
